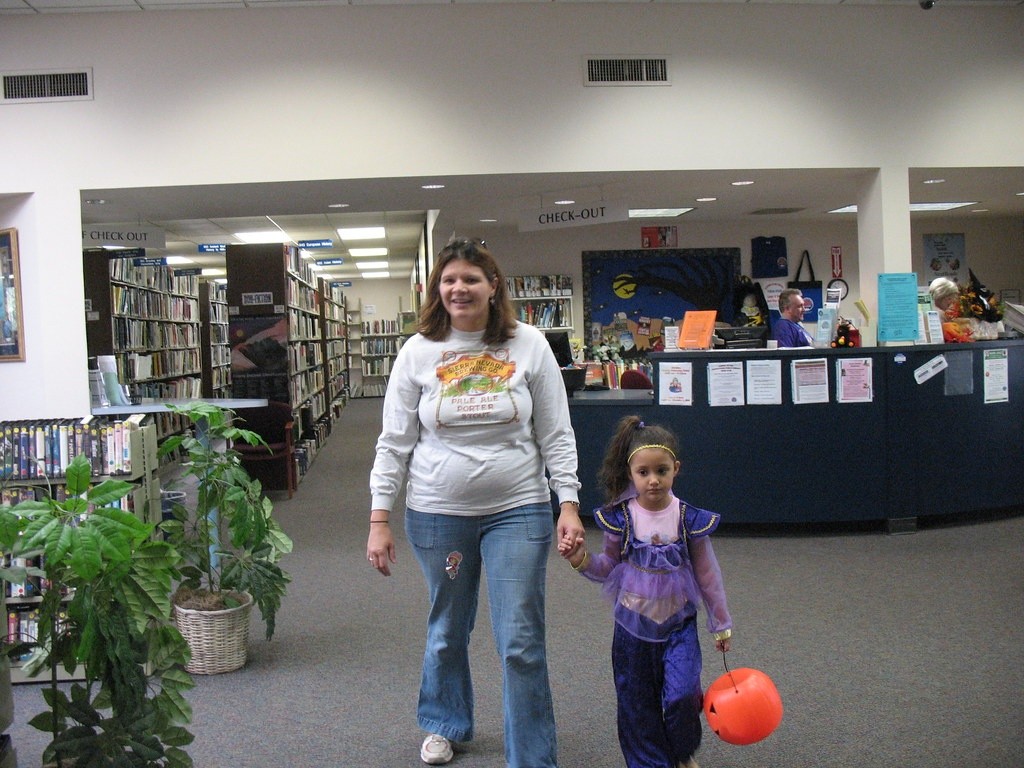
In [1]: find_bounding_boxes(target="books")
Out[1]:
[112,259,232,468]
[501,274,573,327]
[282,244,351,479]
[678,310,717,348]
[350,310,416,397]
[573,360,653,389]
[0,415,155,662]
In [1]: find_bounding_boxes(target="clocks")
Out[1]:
[827,279,849,300]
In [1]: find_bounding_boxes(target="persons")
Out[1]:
[670,378,681,392]
[930,277,959,323]
[737,293,763,327]
[366,236,588,768]
[559,414,732,768]
[773,288,816,348]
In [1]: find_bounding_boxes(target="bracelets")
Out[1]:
[370,521,388,523]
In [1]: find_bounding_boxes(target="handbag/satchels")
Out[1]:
[734,276,771,336]
[788,250,822,324]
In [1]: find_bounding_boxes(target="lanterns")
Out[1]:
[702,668,784,747]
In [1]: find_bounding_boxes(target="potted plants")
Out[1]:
[0,402,294,768]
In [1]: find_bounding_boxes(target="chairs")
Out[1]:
[620,369,653,389]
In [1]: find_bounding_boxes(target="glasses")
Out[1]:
[445,237,487,250]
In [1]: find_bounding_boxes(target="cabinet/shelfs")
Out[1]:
[349,297,416,398]
[84,248,234,477]
[226,244,348,499]
[510,296,574,333]
[0,227,26,363]
[0,424,183,683]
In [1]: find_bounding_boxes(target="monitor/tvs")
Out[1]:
[715,326,767,349]
[543,332,572,368]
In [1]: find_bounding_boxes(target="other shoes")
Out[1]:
[420,733,453,764]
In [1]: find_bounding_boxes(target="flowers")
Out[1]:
[945,269,1009,323]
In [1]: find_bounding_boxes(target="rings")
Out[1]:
[370,558,373,561]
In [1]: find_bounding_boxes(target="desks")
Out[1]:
[90,397,269,567]
